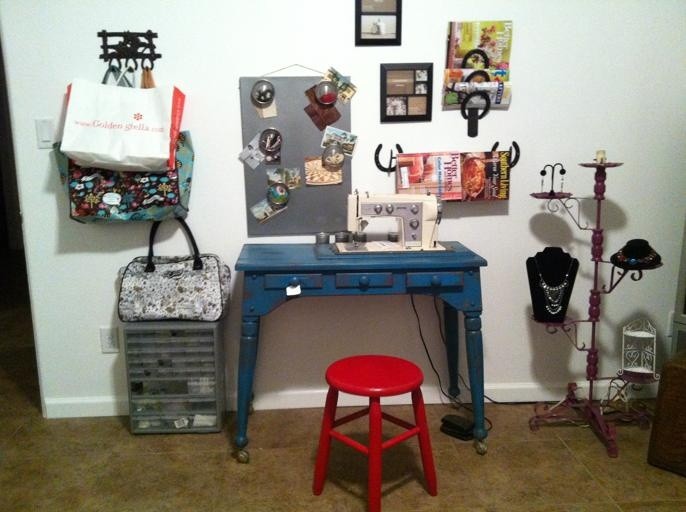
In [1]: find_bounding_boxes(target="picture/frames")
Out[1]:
[380,63,432,123]
[355,0,401,46]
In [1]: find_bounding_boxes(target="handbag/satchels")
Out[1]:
[51,130,195,224]
[60,65,187,172]
[114,217,232,322]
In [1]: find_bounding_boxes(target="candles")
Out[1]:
[596,150,607,162]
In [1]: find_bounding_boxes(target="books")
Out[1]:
[444,151,511,204]
[439,20,513,110]
[395,151,462,202]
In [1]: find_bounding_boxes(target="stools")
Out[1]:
[311,353,438,511]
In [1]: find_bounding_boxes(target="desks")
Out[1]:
[234,243,488,463]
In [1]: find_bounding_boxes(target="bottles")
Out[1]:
[387,232,400,242]
[354,232,367,242]
[316,232,329,244]
[335,232,349,242]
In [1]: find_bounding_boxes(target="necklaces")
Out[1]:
[525,253,574,317]
[614,245,661,265]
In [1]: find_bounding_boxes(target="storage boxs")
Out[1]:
[115,320,224,435]
